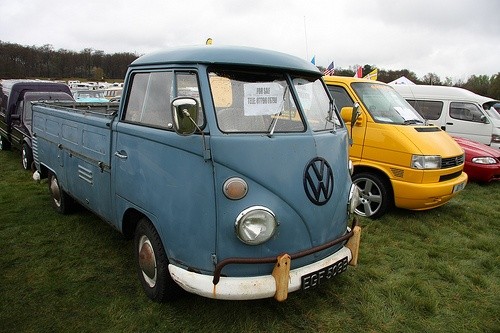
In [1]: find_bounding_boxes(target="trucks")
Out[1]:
[29,45,361,305]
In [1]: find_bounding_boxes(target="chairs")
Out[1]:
[217,105,266,131]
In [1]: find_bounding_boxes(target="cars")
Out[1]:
[56,80,125,95]
[452,135,500,185]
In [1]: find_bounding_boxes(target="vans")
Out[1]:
[387,83,500,149]
[209,77,468,218]
[74,83,90,89]
[0,79,74,170]
